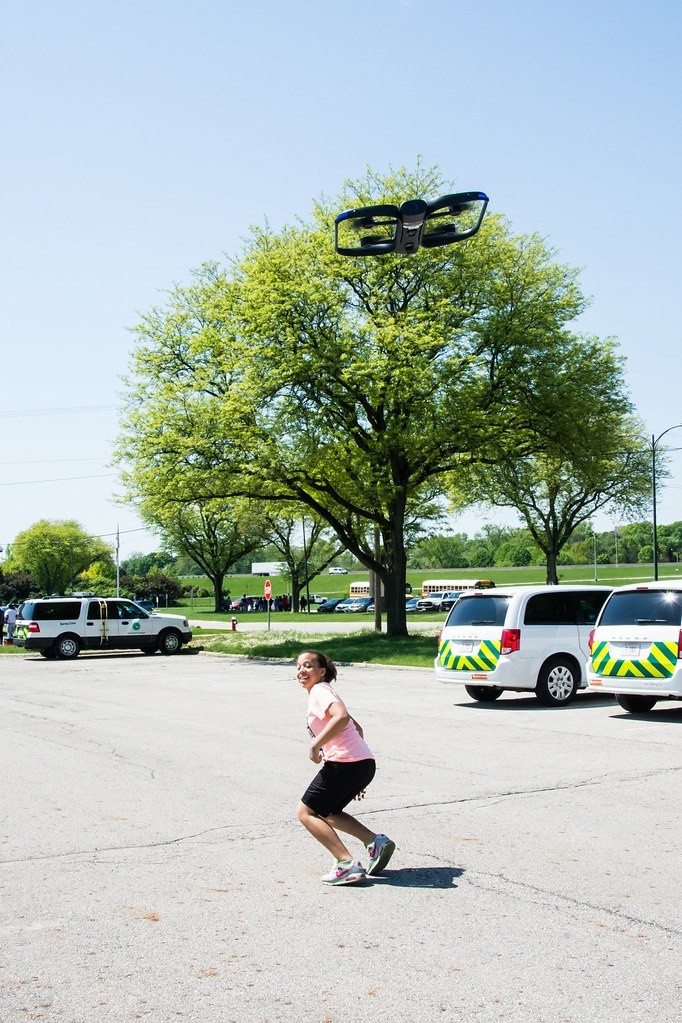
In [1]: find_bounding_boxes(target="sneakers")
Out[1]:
[320,858,366,886]
[365,833,395,875]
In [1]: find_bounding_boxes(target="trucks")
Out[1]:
[299,594,328,604]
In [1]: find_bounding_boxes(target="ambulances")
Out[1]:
[13,592,192,660]
[584,579,682,713]
[434,585,617,708]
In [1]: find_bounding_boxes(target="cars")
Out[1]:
[406,598,423,612]
[0,604,20,612]
[317,598,346,612]
[417,591,466,612]
[335,598,359,612]
[230,596,259,611]
[348,596,374,612]
[366,602,375,612]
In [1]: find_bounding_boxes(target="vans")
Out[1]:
[329,567,348,575]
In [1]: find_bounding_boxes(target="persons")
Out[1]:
[223,596,231,611]
[0,600,23,646]
[295,651,395,886]
[300,596,307,611]
[242,593,291,612]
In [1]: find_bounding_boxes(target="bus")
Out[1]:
[422,580,496,599]
[349,581,414,599]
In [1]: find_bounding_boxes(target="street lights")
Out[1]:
[618,424,682,581]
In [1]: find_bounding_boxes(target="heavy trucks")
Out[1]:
[252,562,297,577]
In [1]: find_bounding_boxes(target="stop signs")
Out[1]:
[265,581,271,600]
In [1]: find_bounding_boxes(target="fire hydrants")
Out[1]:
[231,617,238,630]
